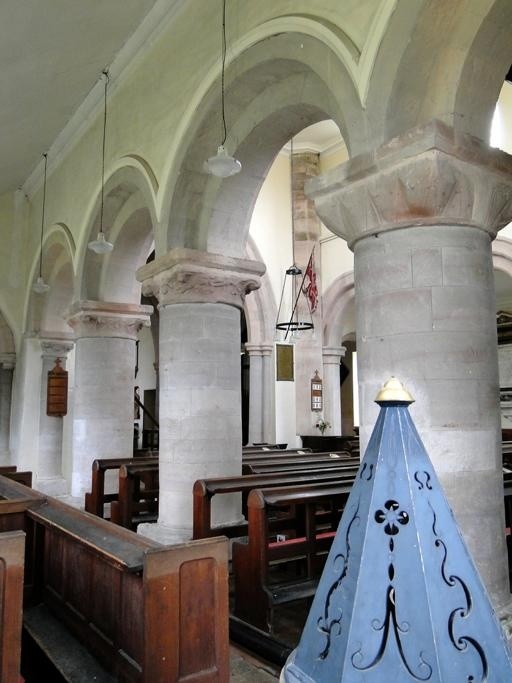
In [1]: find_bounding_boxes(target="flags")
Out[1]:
[304,249,318,314]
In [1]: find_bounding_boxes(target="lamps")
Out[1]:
[29,65,113,299]
[202,2,244,180]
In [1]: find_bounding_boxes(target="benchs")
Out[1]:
[84,446,360,534]
[502,426,512,592]
[193,470,362,667]
